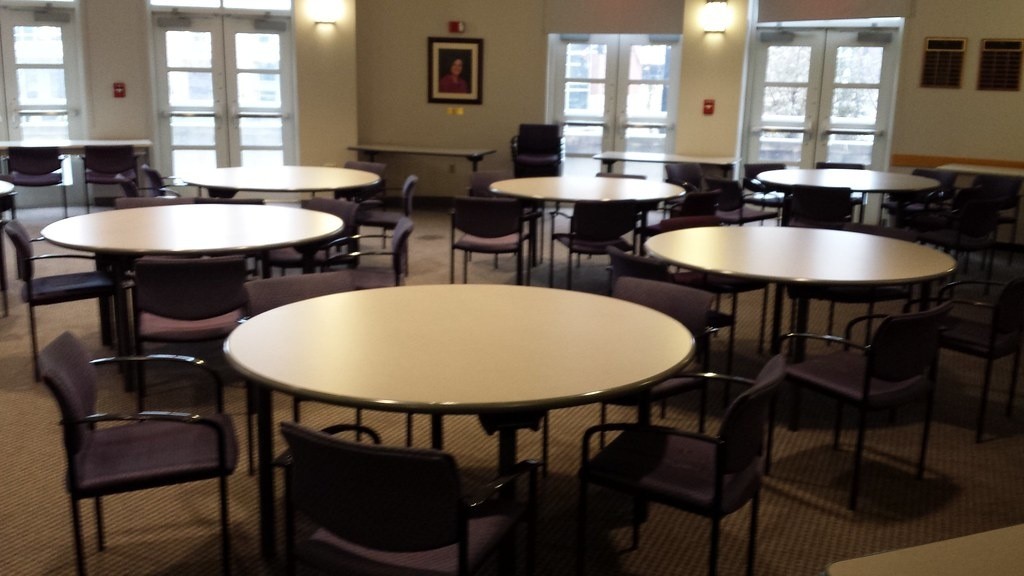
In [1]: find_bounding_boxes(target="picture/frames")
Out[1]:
[428,36,485,105]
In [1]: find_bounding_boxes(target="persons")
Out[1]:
[439,56,469,93]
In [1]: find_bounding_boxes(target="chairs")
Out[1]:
[0,124,1024,576]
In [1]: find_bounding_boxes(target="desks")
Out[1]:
[489,175,686,286]
[936,162,1024,194]
[645,225,960,430]
[0,179,16,292]
[347,144,496,210]
[593,151,740,177]
[1,139,153,185]
[40,203,345,390]
[180,165,381,201]
[758,169,940,223]
[222,284,696,576]
